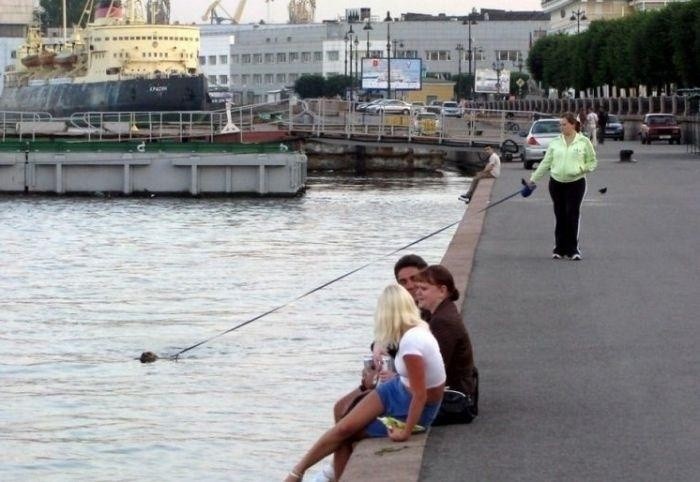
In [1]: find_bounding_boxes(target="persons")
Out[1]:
[322,265,479,479]
[526,111,598,260]
[458,144,501,203]
[576,105,610,145]
[284,284,447,482]
[333,254,428,424]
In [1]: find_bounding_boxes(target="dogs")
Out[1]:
[140,352,158,363]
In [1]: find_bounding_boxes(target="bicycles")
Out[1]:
[499,119,519,134]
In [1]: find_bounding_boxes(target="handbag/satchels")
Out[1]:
[431,389,473,425]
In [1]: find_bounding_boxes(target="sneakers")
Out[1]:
[458,195,469,202]
[552,252,582,260]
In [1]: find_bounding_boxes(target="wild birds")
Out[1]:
[599,188,607,193]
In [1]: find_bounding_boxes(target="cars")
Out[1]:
[637,113,681,145]
[356,95,462,132]
[595,114,624,141]
[520,118,563,169]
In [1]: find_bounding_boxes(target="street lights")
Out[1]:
[455,13,528,94]
[569,8,588,36]
[341,11,404,101]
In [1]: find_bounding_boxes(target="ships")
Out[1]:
[0,0,213,130]
